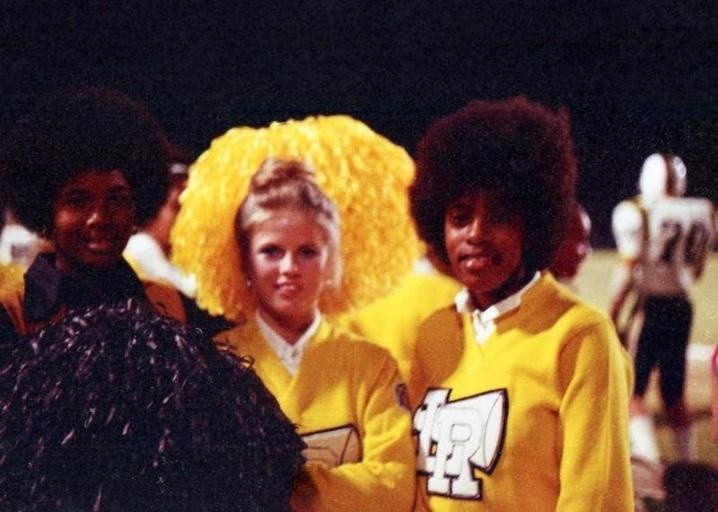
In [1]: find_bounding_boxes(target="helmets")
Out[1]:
[639,152,688,196]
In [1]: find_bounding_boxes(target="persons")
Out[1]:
[552,205,594,281]
[125,164,228,336]
[406,89,640,512]
[0,84,220,402]
[200,160,420,512]
[1,162,44,266]
[604,150,718,500]
